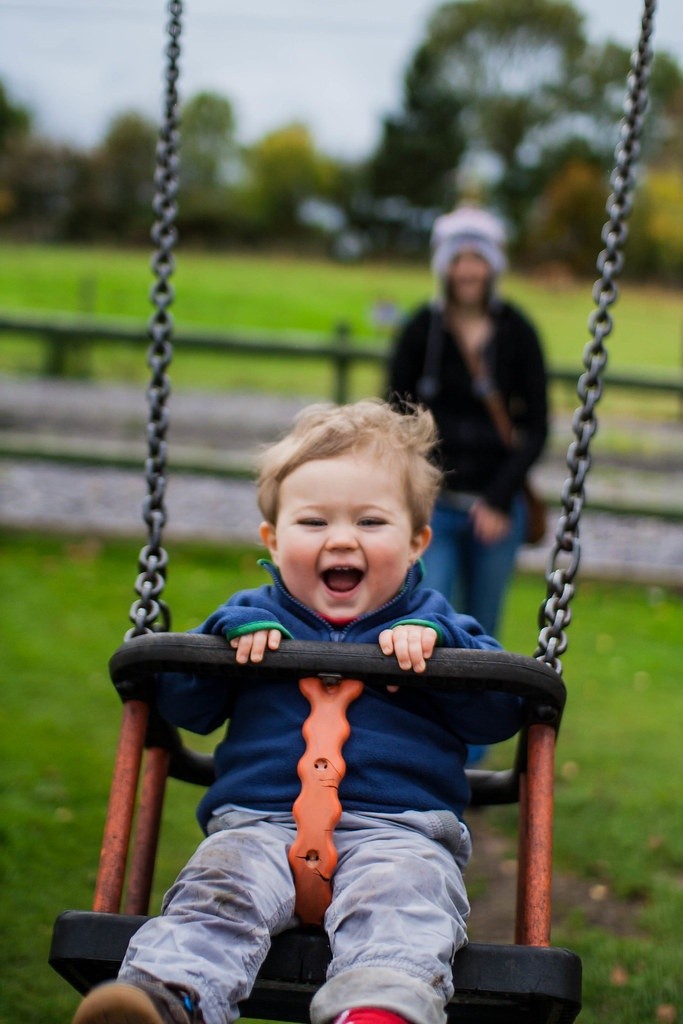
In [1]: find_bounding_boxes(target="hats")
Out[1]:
[431,209,509,273]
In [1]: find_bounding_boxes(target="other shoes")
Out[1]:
[72,982,179,1024]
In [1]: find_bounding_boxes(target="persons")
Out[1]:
[369,208,553,772]
[69,394,519,1024]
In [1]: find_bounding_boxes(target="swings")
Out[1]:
[51,5,657,1023]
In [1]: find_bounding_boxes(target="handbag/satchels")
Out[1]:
[527,497,546,543]
